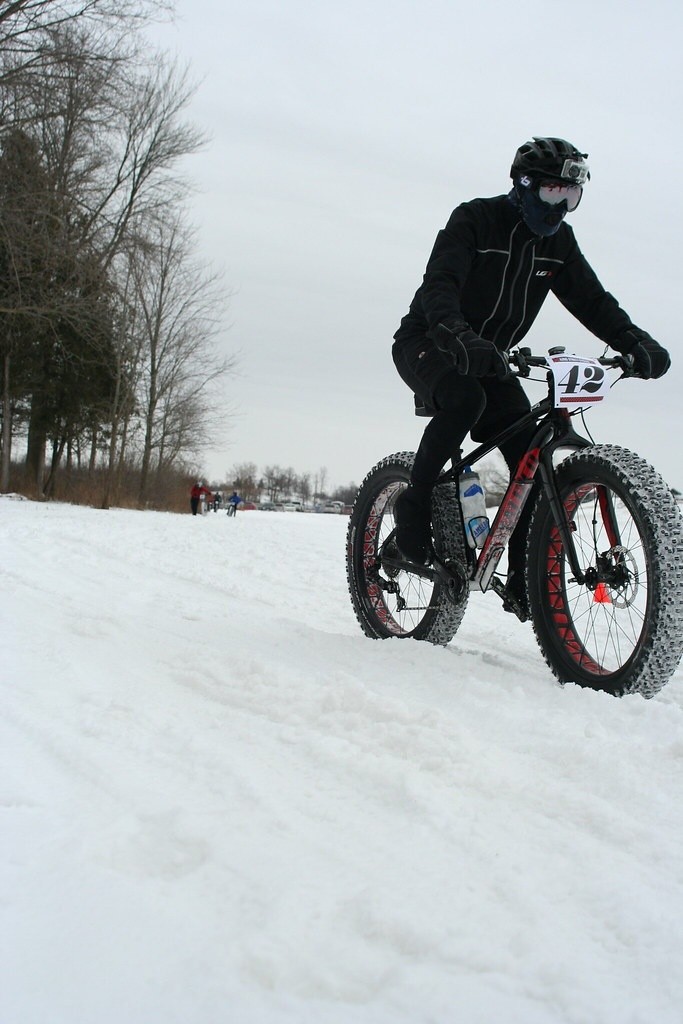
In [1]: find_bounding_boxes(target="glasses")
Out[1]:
[536,185,583,213]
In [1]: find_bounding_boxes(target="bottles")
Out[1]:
[458,466,489,548]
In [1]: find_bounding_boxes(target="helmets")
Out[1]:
[510,136,591,185]
[196,482,203,490]
[232,492,237,496]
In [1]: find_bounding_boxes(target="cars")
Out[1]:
[237,499,355,515]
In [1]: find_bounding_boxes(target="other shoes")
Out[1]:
[394,481,432,563]
[503,568,529,613]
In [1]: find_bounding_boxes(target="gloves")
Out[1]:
[611,327,671,380]
[447,326,505,378]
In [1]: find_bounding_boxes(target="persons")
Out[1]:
[227,491,241,518]
[391,137,671,620]
[190,482,212,515]
[205,491,222,513]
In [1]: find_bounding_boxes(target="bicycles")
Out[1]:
[227,499,239,517]
[213,499,223,513]
[343,346,683,702]
[196,493,211,515]
